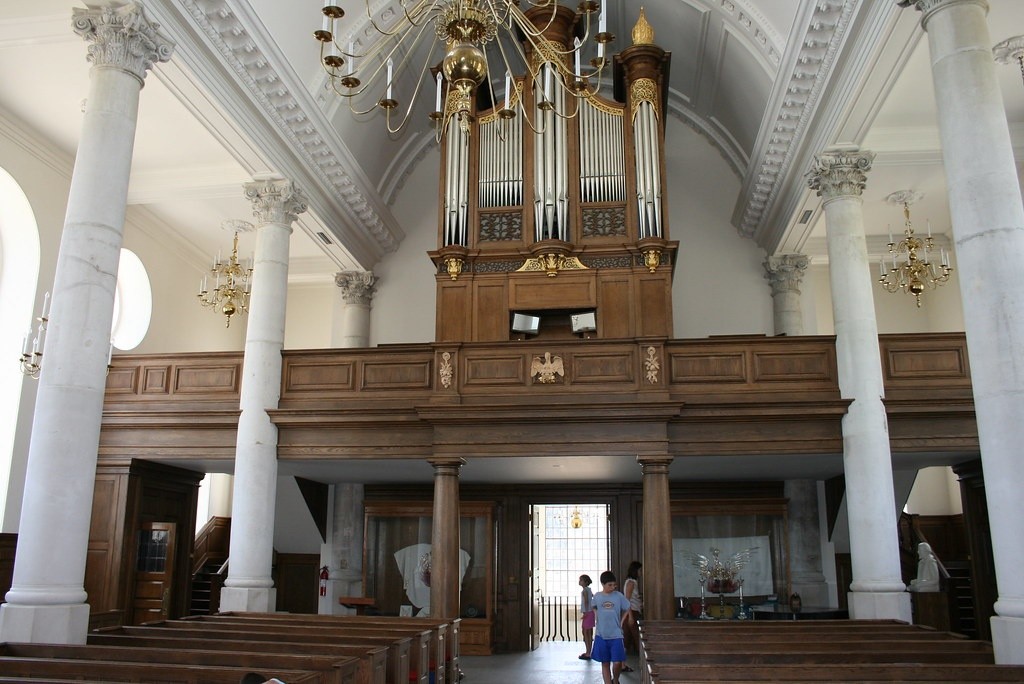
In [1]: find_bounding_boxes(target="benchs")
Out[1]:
[637,618,1024,684]
[0,613,467,683]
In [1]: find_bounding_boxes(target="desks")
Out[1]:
[340,596,376,615]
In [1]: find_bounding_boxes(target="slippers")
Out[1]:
[579,653,590,659]
[621,666,634,672]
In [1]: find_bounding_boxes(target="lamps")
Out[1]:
[198,219,256,328]
[313,0,617,146]
[878,188,952,305]
[19,291,115,379]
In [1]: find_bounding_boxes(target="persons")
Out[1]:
[579,561,643,684]
[906,542,940,592]
